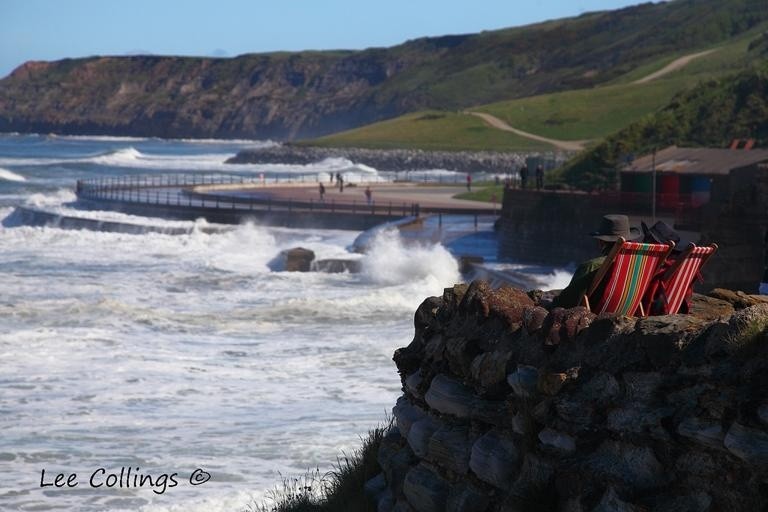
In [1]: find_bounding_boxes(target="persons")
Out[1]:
[466,176,472,190]
[365,186,372,203]
[319,172,343,200]
[554,214,681,306]
[520,164,544,189]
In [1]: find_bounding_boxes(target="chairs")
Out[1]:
[582,236,719,318]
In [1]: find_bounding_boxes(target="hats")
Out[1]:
[590,214,639,242]
[640,218,681,244]
